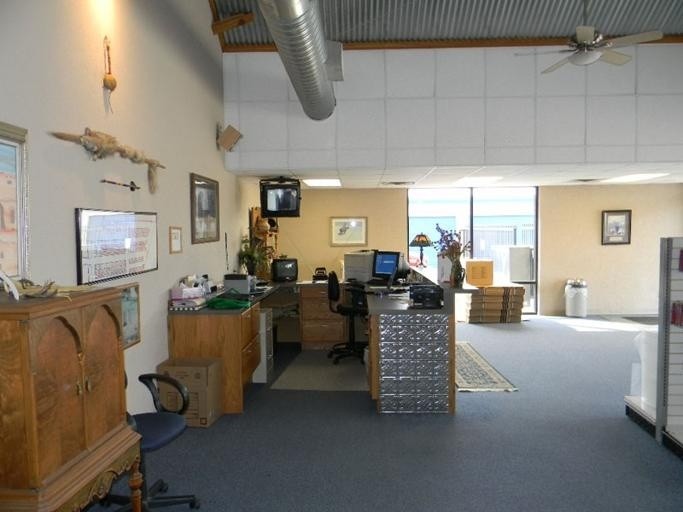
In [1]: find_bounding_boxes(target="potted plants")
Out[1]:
[238,234,274,277]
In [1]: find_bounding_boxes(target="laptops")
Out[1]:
[365,266,397,292]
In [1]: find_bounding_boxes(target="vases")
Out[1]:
[449,261,466,289]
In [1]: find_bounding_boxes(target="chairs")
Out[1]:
[99,374,195,512]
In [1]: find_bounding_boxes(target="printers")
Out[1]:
[223,273,251,295]
[344,250,374,283]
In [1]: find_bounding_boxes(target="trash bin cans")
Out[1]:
[565,279,587,318]
[364,346,369,376]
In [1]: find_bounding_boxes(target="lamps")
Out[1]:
[409,232,433,267]
[569,51,601,66]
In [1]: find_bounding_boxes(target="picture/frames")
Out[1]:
[329,216,369,248]
[190,172,221,244]
[0,120,31,283]
[115,282,141,347]
[73,206,157,285]
[601,209,632,245]
[169,225,183,253]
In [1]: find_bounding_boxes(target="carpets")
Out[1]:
[454,340,517,391]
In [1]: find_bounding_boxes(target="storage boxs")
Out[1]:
[157,355,224,429]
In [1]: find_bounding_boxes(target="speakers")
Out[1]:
[216,125,241,151]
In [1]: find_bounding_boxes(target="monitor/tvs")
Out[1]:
[372,251,400,278]
[271,259,298,282]
[261,184,300,218]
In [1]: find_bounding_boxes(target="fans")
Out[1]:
[515,0,662,78]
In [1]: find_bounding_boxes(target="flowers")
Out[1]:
[430,223,473,261]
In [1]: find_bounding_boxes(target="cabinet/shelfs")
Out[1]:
[298,286,350,352]
[0,287,148,512]
[167,302,261,411]
[363,293,454,414]
[252,308,274,384]
